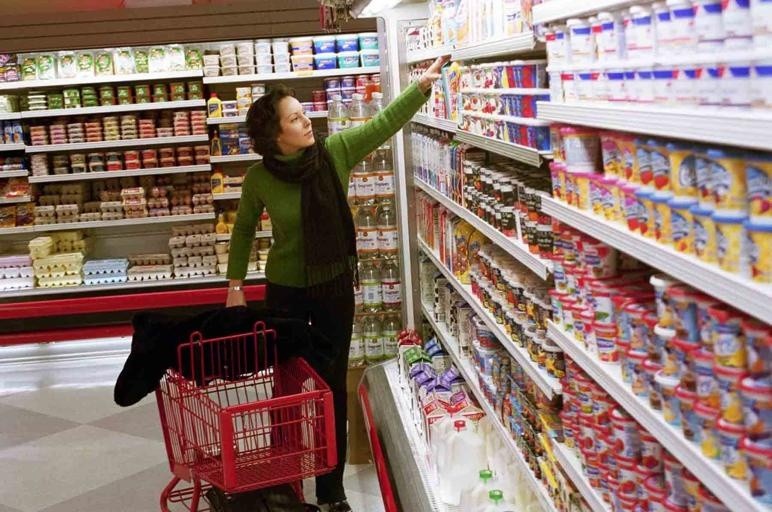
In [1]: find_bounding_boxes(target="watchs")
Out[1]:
[228,286,244,291]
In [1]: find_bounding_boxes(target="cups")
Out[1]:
[456,58,563,479]
[545,122,772,512]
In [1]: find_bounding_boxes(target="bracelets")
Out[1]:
[418,77,432,90]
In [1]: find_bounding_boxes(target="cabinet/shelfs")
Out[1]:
[201,65,382,282]
[0,69,218,302]
[398,2,771,511]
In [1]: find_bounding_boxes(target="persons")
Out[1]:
[224,55,451,512]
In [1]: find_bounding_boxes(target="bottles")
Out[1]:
[217,209,226,235]
[211,131,222,155]
[208,93,223,119]
[430,409,546,512]
[212,166,225,192]
[327,92,403,367]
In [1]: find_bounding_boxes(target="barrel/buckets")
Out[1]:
[325,92,403,369]
[429,398,549,512]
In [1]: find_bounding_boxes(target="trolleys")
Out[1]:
[132,303,351,512]
